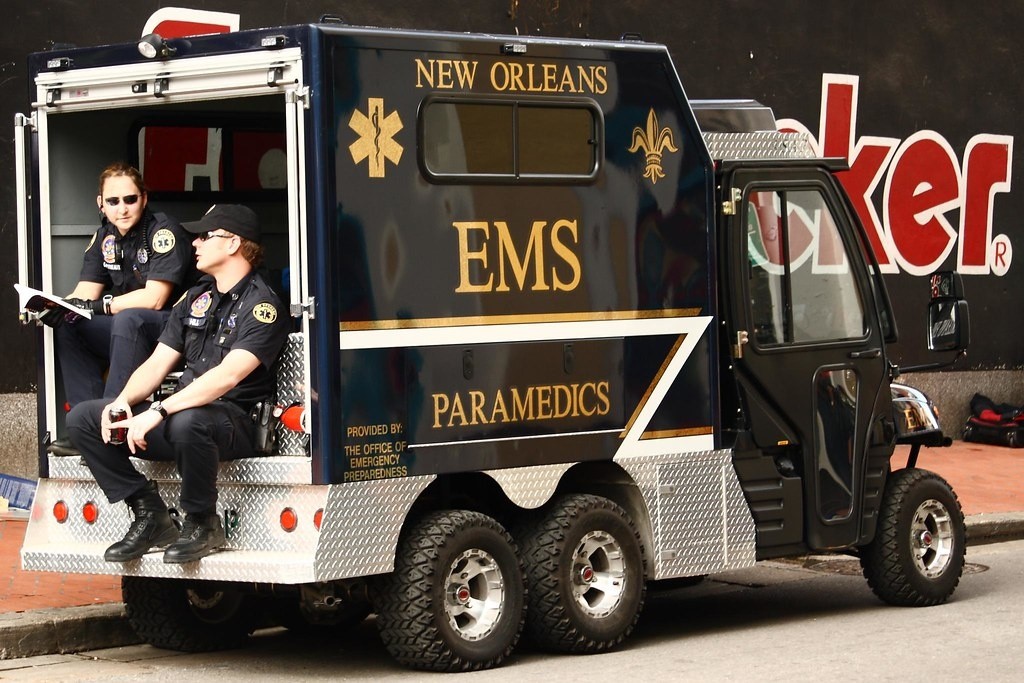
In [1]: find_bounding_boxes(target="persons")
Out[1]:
[36,164,199,467]
[65,200,290,564]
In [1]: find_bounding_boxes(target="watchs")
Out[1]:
[150,400,168,420]
[103,294,113,315]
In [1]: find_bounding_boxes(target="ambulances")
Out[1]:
[15,14,968,672]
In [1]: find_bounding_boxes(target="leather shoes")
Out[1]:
[48,436,81,455]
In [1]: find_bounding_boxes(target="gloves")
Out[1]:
[60,298,106,326]
[38,309,64,329]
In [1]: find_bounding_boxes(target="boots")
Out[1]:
[103,480,179,561]
[163,512,225,563]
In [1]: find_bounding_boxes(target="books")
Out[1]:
[12,281,92,328]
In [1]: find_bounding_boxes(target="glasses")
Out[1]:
[104,194,143,207]
[199,231,233,241]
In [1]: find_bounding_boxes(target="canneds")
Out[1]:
[109,408,127,445]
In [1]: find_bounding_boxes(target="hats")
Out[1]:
[179,203,263,244]
